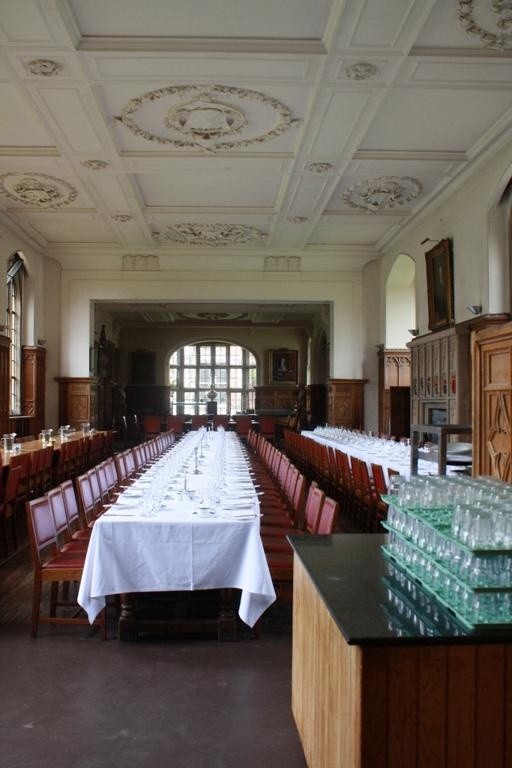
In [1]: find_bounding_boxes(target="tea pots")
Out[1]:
[1,423,90,452]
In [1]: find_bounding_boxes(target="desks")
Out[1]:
[285,534,512,768]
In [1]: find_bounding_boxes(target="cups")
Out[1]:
[316,423,431,464]
[384,474,512,626]
[143,423,225,517]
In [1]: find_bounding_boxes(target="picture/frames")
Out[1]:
[425,237,455,331]
[264,348,299,386]
[128,350,157,384]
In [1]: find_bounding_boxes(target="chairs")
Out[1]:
[0,415,485,645]
